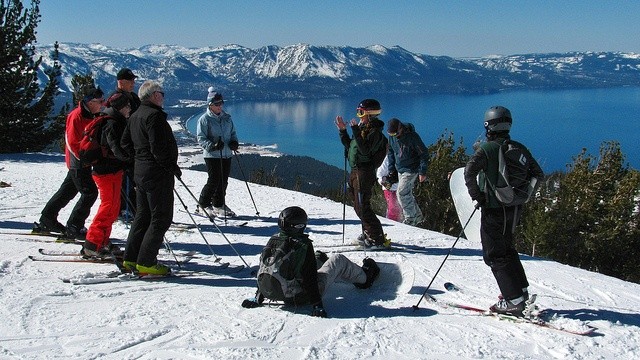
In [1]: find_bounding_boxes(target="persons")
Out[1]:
[119,79,182,276]
[375,144,402,223]
[464,106,544,313]
[107,67,141,222]
[195,84,239,218]
[241,206,380,318]
[333,99,392,251]
[387,117,432,224]
[38,88,106,240]
[80,92,132,259]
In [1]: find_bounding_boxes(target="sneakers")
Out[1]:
[121,209,134,223]
[489,295,525,316]
[212,204,236,220]
[39,217,65,232]
[358,235,391,249]
[415,209,423,224]
[358,231,387,240]
[403,216,416,226]
[104,241,124,258]
[82,241,116,262]
[136,261,171,277]
[64,221,88,239]
[122,259,138,273]
[195,204,215,217]
[498,287,529,302]
[352,258,380,289]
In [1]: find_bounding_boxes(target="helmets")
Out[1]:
[359,99,381,116]
[278,206,307,233]
[484,106,512,132]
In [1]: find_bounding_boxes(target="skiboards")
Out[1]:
[178,208,273,227]
[58,263,246,285]
[425,282,598,335]
[0,231,83,245]
[313,243,426,254]
[169,221,248,232]
[28,248,196,264]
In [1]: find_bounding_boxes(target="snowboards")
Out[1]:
[449,167,482,242]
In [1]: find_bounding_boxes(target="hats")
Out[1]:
[79,86,105,103]
[387,117,403,134]
[207,86,222,110]
[116,68,137,80]
[109,92,128,109]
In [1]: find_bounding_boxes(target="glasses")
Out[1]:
[125,103,131,108]
[388,132,400,137]
[91,99,106,103]
[212,100,225,106]
[153,90,164,97]
[357,107,366,117]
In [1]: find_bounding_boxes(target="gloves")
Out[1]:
[213,139,224,150]
[381,181,391,190]
[168,165,181,177]
[310,303,328,318]
[241,298,258,308]
[228,139,239,150]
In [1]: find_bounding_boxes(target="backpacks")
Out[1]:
[482,137,534,206]
[80,113,122,171]
[257,232,313,307]
